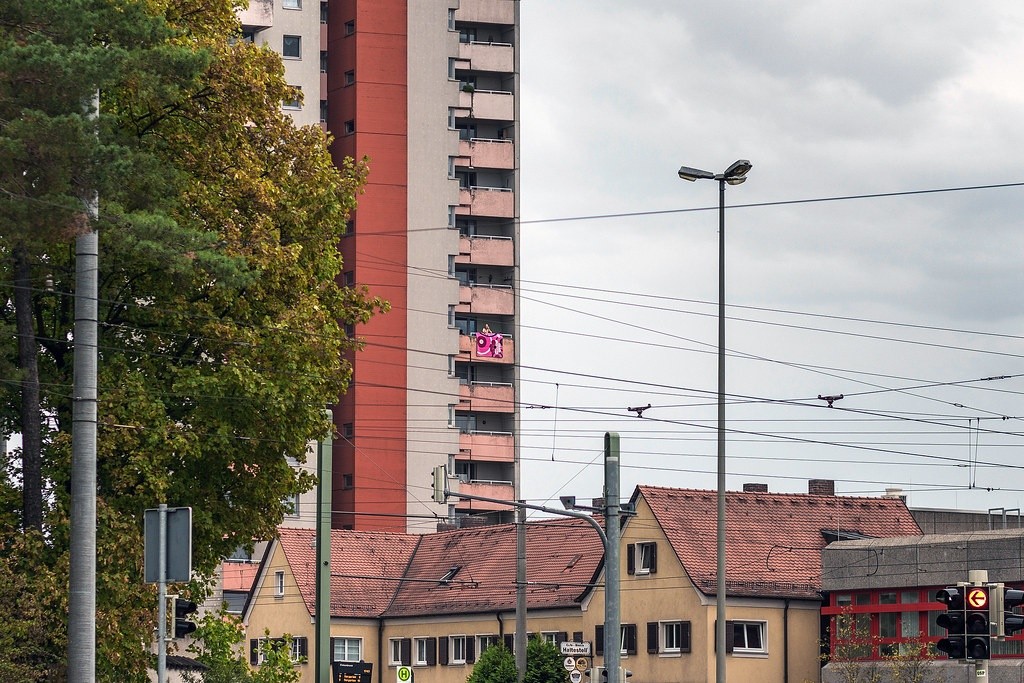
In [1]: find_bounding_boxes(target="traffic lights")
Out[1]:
[585,666,607,683]
[171,598,196,639]
[430,466,445,502]
[935,582,1024,660]
[619,666,632,683]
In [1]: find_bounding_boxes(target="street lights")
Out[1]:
[678,160,753,683]
